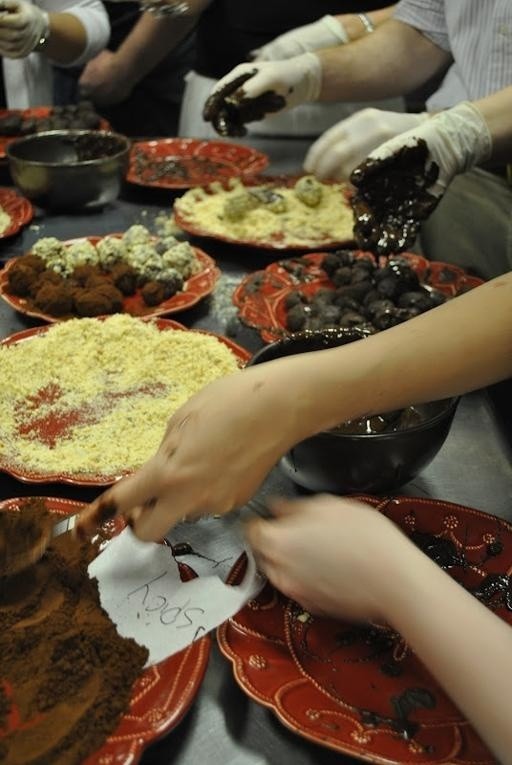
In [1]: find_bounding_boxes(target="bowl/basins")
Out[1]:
[9,127,129,211]
[245,327,461,496]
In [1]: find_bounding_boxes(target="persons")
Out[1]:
[0,0,111,113]
[75,271,512,765]
[202,1,512,282]
[80,0,406,173]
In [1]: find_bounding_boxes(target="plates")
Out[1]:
[125,137,268,193]
[2,315,251,491]
[1,109,108,157]
[0,495,214,765]
[234,258,486,341]
[1,188,34,240]
[1,234,222,323]
[174,175,359,251]
[212,494,512,765]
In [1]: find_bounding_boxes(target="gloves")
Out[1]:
[0,0,50,59]
[348,100,493,256]
[298,106,429,180]
[203,51,323,138]
[249,14,349,63]
